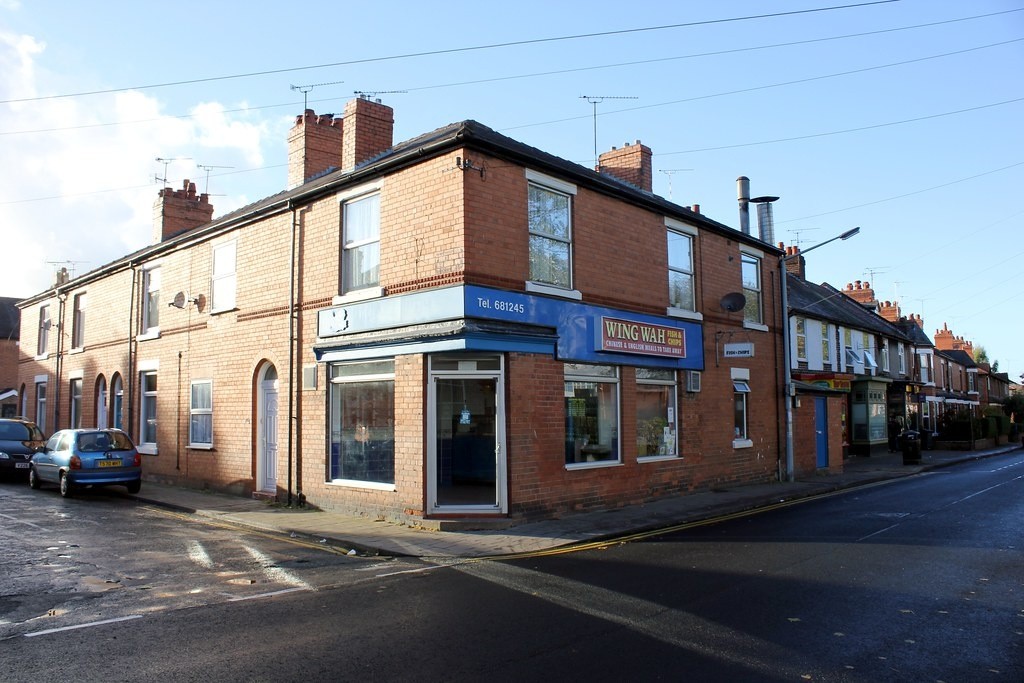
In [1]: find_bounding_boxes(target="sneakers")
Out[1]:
[893,450,896,452]
[888,449,891,452]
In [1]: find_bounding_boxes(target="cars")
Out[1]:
[0,415,48,477]
[28,427,143,498]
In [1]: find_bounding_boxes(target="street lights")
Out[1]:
[778,225,863,481]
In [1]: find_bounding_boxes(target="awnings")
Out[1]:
[846,349,864,364]
[865,351,878,367]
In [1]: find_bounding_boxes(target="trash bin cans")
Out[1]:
[902,430,922,465]
[1007,423,1020,442]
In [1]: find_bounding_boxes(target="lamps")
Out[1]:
[172,289,201,310]
[721,292,746,312]
[41,318,61,331]
